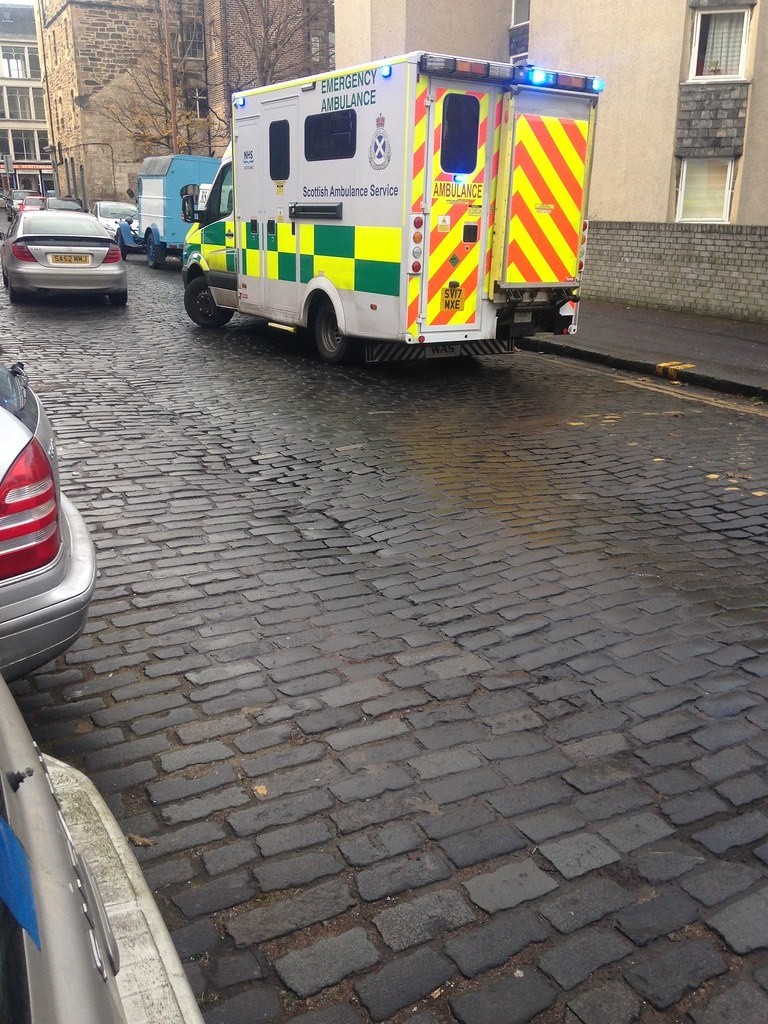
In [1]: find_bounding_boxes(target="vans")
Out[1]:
[197,182,233,214]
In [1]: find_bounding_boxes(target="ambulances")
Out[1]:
[180,49,606,366]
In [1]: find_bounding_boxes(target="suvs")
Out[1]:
[5,189,40,222]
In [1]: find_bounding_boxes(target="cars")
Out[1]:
[113,155,224,267]
[0,358,100,684]
[17,196,47,213]
[0,678,207,1024]
[89,200,139,238]
[40,196,89,212]
[0,211,129,306]
